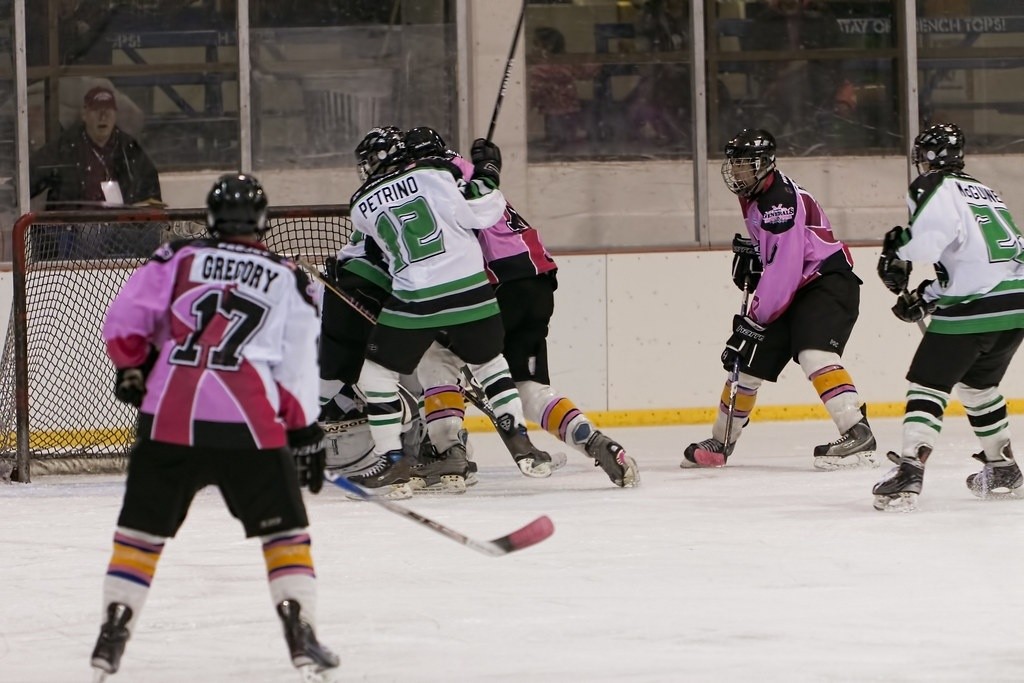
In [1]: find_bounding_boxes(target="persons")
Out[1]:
[0,88,164,263]
[680,128,879,471]
[317,127,641,501]
[872,124,1023,511]
[91,173,342,683]
[528,26,882,155]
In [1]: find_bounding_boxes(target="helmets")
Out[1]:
[721,128,775,198]
[912,124,966,169]
[405,127,447,158]
[355,125,411,179]
[206,172,265,236]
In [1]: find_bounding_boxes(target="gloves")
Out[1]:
[722,314,768,372]
[731,233,764,293]
[114,364,146,405]
[891,279,938,323]
[472,138,502,186]
[286,421,323,494]
[877,226,912,295]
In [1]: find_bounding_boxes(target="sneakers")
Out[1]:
[964,440,1024,499]
[345,449,412,501]
[90,601,132,682]
[874,442,933,512]
[413,429,469,494]
[496,413,566,477]
[680,438,736,468]
[572,421,641,486]
[278,599,339,683]
[814,403,880,470]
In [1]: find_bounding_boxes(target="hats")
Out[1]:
[84,87,117,113]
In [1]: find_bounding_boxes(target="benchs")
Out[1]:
[0,0,1024,177]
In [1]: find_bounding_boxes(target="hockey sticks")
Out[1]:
[903,285,927,336]
[684,275,751,466]
[295,254,496,421]
[461,365,499,431]
[486,0,529,142]
[323,469,555,558]
